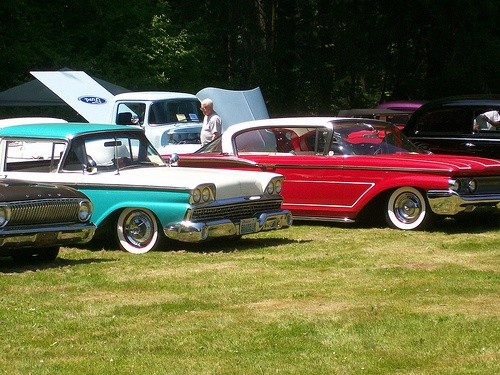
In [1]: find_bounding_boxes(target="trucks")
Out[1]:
[28,69,277,157]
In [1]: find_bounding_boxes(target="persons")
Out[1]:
[199,99,222,152]
[473,110,500,133]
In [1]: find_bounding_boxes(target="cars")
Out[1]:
[402,96,500,162]
[1,116,129,167]
[0,176,97,266]
[159,115,500,231]
[0,123,294,255]
[335,99,428,144]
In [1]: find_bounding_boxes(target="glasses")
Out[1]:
[200,105,213,111]
